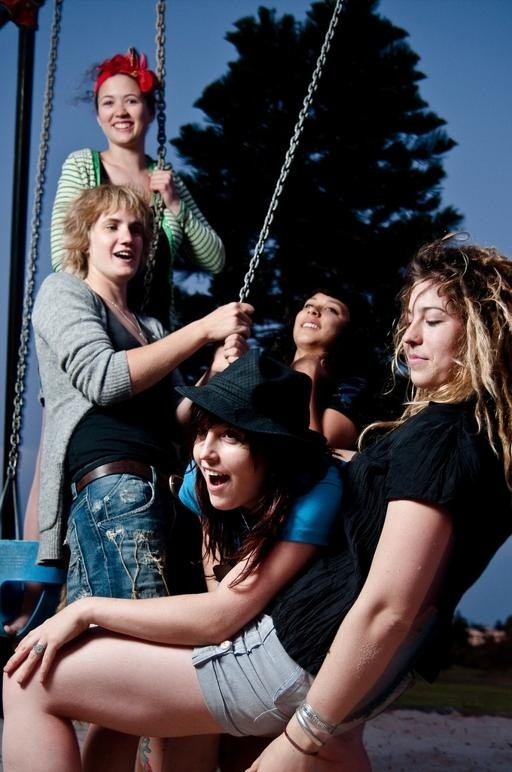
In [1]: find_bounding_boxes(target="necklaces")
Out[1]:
[84,279,149,345]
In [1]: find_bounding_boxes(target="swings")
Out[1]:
[0,1,360,637]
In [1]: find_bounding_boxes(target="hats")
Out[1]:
[173,350,332,441]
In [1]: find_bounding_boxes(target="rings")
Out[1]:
[33,643,45,654]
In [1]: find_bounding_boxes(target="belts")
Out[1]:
[74,461,153,493]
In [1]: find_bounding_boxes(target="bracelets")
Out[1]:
[293,708,324,747]
[301,701,337,736]
[284,727,319,756]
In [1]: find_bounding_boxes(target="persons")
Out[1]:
[3,353,374,772]
[1,230,512,772]
[268,286,380,449]
[0,46,227,637]
[32,179,255,772]
